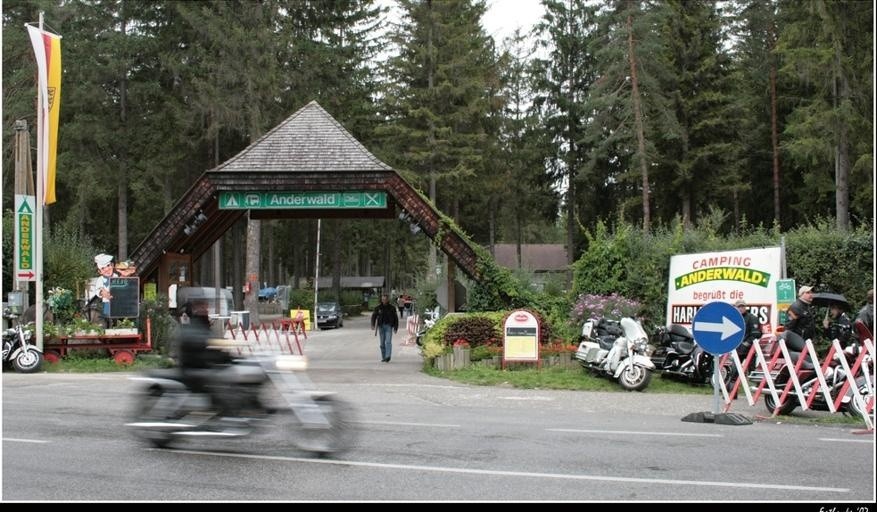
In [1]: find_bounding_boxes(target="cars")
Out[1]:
[308,301,344,331]
[258,286,277,303]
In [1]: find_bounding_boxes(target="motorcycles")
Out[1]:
[126,355,362,454]
[760,351,876,414]
[572,313,768,387]
[2,313,45,375]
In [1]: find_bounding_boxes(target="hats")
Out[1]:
[798,285,815,296]
[94,253,114,268]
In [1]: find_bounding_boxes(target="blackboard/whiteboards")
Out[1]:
[108,277,139,318]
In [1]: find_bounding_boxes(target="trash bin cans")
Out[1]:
[230,311,248,328]
[210,316,230,338]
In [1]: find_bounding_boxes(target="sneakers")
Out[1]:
[382,357,390,362]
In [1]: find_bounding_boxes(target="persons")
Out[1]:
[92,253,136,328]
[370,294,399,363]
[395,294,417,319]
[853,288,873,368]
[822,304,852,360]
[731,299,762,380]
[782,285,824,367]
[172,281,281,436]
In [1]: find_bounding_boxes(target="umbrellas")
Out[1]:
[810,291,853,319]
